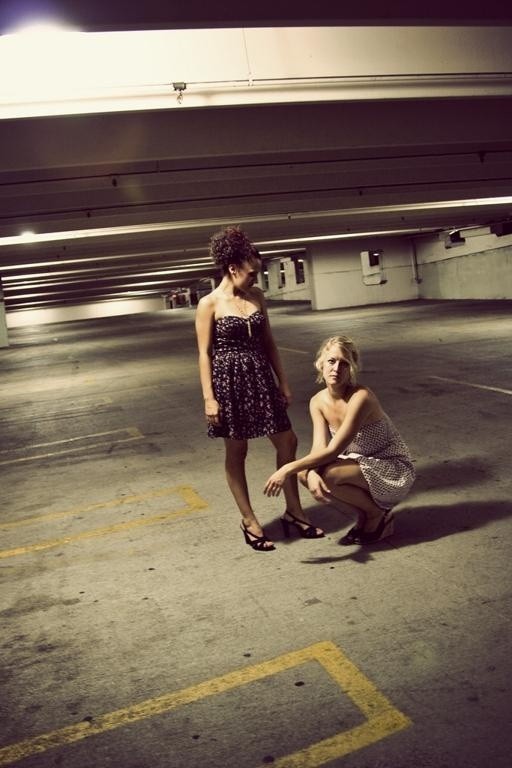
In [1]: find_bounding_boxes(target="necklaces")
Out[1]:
[219,285,254,339]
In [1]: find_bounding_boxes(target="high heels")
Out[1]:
[279,510,324,538]
[239,518,276,551]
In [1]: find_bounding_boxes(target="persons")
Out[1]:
[194,223,326,552]
[261,333,418,545]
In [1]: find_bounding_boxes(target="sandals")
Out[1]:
[345,527,364,545]
[352,508,394,545]
